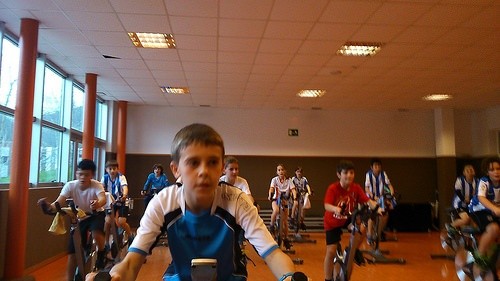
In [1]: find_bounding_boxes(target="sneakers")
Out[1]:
[284,237,291,249]
[96,252,107,270]
[469,250,490,270]
[300,223,306,230]
[345,246,365,266]
[127,235,134,248]
[444,222,456,239]
[366,234,373,245]
[270,225,275,233]
[105,245,110,253]
[291,216,295,224]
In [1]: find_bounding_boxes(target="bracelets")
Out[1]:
[492,205,496,213]
[279,272,294,281]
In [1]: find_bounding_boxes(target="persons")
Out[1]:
[446,156,500,281]
[85,123,311,281]
[323,160,395,281]
[46,158,132,281]
